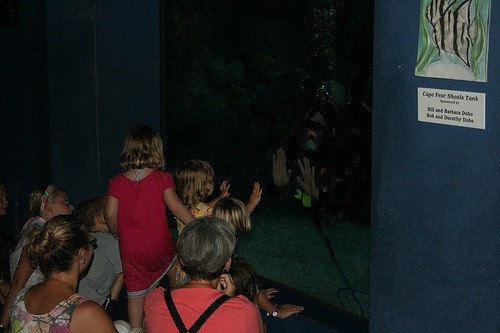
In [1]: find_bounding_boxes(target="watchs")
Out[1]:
[0,324,9,329]
[272,306,279,317]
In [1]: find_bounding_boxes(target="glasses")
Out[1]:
[78,234,97,253]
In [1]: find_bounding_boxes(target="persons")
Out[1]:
[104,126,197,333]
[142,216,267,333]
[174,160,263,287]
[210,197,303,320]
[0,185,75,333]
[76,197,128,321]
[9,214,143,333]
[258,100,372,227]
